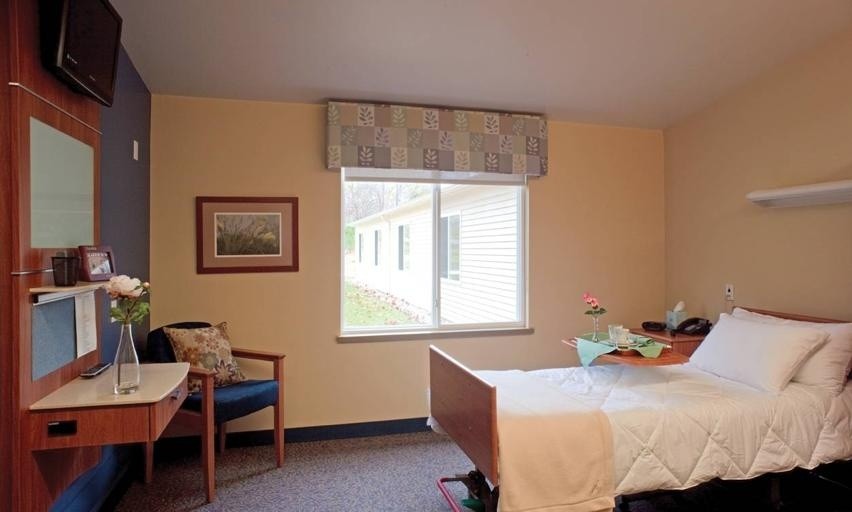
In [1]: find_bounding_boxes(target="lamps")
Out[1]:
[744,178,852,210]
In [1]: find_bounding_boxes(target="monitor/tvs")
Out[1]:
[55,0,123,108]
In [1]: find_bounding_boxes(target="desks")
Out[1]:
[560,331,689,369]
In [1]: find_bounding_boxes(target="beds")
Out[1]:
[429,304,852,511]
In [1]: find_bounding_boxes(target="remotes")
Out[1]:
[80,362,112,377]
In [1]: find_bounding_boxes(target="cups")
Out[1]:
[607,323,630,351]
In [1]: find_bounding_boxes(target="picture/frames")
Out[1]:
[195,195,299,275]
[78,244,118,282]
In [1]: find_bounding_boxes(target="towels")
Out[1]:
[577,331,668,367]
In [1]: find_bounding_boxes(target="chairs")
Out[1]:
[143,322,286,502]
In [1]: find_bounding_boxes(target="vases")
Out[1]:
[592,314,602,343]
[112,321,141,395]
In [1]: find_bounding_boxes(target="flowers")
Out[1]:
[583,292,608,316]
[102,275,153,387]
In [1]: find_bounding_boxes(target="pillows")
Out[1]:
[733,306,852,396]
[161,321,247,396]
[688,312,832,394]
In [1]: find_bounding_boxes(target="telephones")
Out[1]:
[678,317,712,336]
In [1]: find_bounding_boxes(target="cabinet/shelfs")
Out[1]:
[0,81,191,512]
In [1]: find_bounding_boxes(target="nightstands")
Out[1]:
[628,321,706,358]
